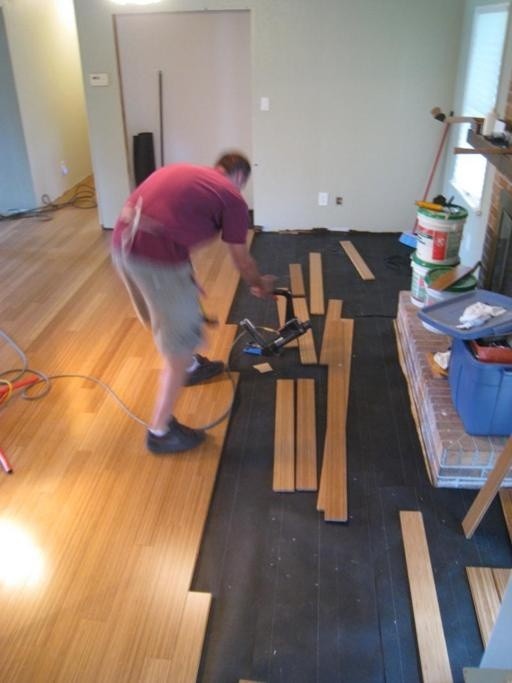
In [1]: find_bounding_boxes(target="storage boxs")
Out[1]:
[448,338,511,435]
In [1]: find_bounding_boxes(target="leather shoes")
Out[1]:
[182,352,224,387]
[147,415,205,452]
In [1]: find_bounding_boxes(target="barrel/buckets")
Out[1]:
[415,203,468,262]
[409,252,461,305]
[420,267,478,334]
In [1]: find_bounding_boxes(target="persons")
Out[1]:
[110,152,276,456]
